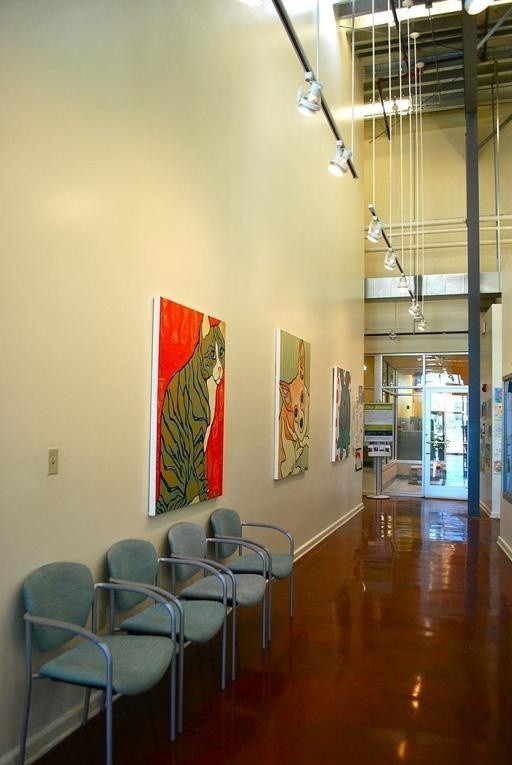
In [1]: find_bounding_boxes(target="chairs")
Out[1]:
[169,521,266,684]
[17,560,178,765]
[102,538,229,734]
[210,507,294,642]
